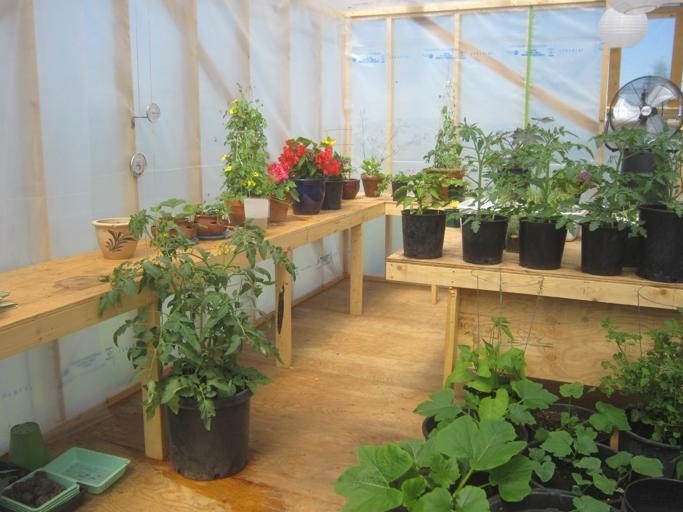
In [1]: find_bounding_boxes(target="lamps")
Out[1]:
[599,8,650,46]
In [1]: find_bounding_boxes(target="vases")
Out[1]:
[8,422,51,468]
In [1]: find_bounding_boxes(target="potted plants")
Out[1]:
[95,200,296,481]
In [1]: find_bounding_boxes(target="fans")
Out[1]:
[601,74,682,151]
